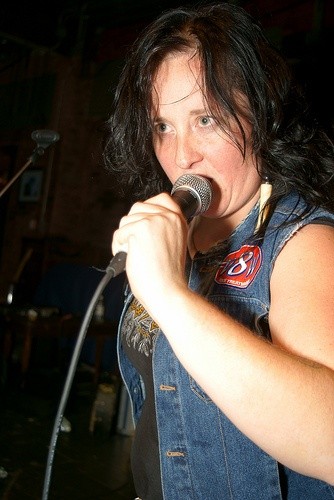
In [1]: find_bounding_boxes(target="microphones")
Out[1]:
[106,175,215,277]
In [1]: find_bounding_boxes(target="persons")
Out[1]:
[102,0,334,500]
